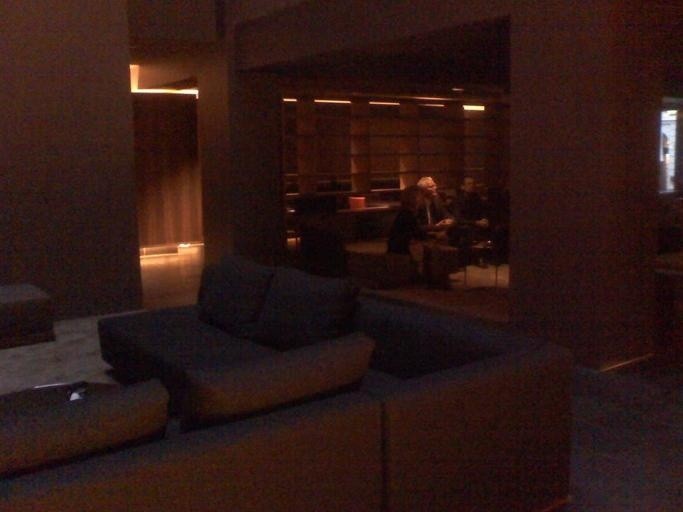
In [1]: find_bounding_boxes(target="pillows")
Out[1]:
[197,262,271,340]
[180,331,376,429]
[252,264,361,354]
[0,377,170,479]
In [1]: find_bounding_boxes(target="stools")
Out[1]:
[0,283,57,350]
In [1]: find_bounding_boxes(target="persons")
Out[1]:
[389,183,452,289]
[417,176,457,244]
[449,174,508,267]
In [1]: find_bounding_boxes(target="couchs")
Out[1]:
[0,262,578,512]
